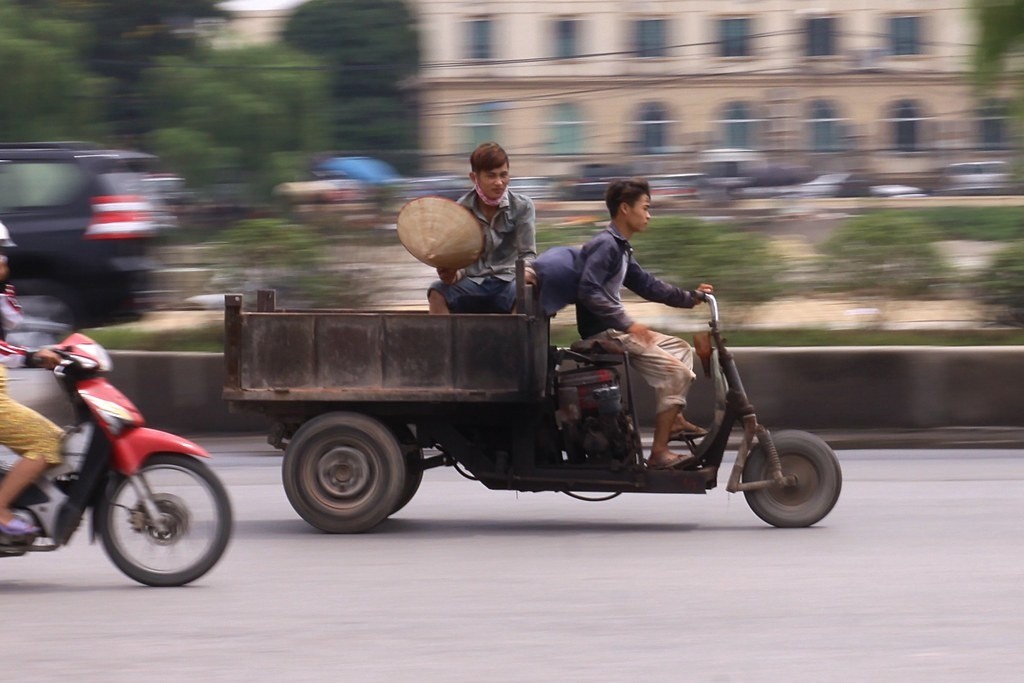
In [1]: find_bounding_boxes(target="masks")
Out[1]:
[472,173,508,206]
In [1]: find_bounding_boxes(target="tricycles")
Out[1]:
[215,253,844,537]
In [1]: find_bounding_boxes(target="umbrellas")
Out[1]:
[311,155,406,185]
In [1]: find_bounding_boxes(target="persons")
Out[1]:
[0,221,63,537]
[425,139,539,317]
[574,176,716,469]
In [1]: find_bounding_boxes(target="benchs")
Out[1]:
[549,340,628,370]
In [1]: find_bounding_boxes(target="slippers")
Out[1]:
[0,518,39,534]
[670,427,710,444]
[650,454,695,470]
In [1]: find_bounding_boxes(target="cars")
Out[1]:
[309,146,1019,208]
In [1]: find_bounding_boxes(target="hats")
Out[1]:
[397,196,484,271]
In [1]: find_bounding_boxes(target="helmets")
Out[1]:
[0,221,18,247]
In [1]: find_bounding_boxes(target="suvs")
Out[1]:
[0,140,185,330]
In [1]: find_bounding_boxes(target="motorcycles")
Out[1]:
[1,326,236,586]
